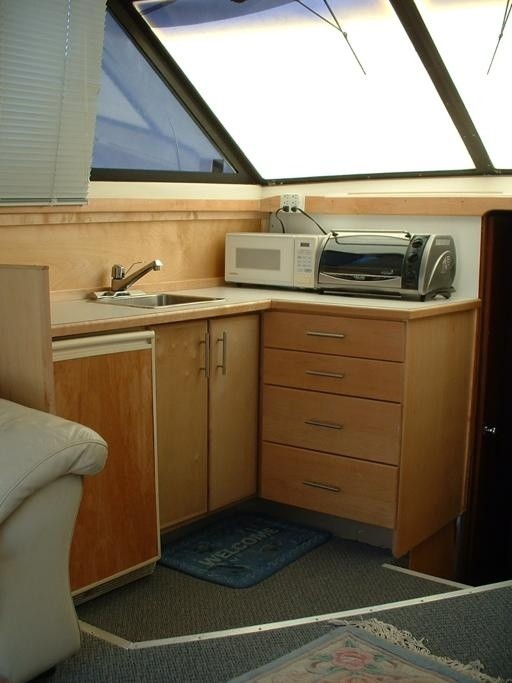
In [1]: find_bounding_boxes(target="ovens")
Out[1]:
[316,229,457,302]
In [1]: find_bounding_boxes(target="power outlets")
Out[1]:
[280,193,305,213]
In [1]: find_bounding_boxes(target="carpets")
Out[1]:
[227,616,512,683]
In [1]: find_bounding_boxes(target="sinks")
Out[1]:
[90,290,226,310]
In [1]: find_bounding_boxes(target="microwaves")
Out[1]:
[224,232,327,289]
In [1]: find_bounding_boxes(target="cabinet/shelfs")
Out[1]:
[147,313,261,547]
[55,329,162,603]
[261,311,481,577]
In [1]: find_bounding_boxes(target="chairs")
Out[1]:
[0,398,108,683]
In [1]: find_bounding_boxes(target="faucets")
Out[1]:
[110,258,162,291]
